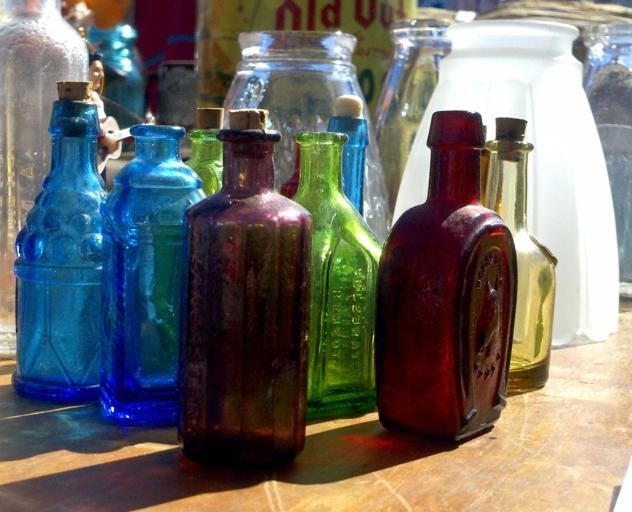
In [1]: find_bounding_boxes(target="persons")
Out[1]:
[89,52,117,154]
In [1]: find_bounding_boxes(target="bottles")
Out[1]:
[221,32,374,192]
[11,81,117,405]
[99,125,208,429]
[388,21,620,349]
[180,107,312,470]
[374,111,518,446]
[0,0,89,353]
[486,117,558,393]
[292,131,383,421]
[579,20,632,305]
[327,94,369,224]
[372,25,455,226]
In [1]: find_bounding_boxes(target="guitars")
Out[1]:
[98,112,155,175]
